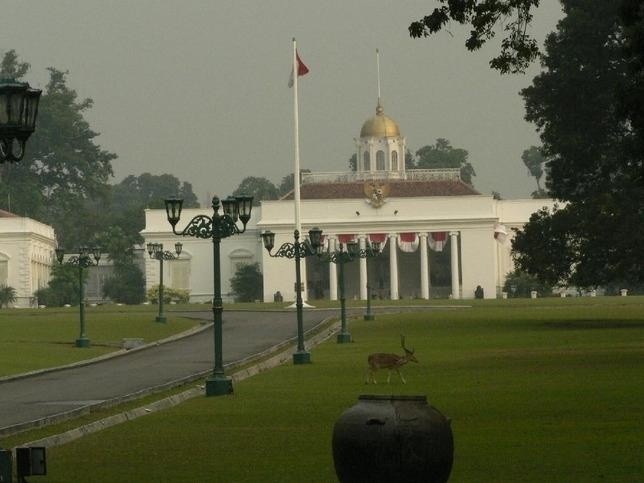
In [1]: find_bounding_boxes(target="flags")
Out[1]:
[287,47,308,88]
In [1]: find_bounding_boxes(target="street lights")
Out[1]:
[260,227,326,364]
[348,237,382,320]
[164,195,255,394]
[1,77,44,162]
[315,241,360,344]
[56,245,104,348]
[146,242,183,323]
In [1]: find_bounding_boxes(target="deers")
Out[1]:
[365,334,419,385]
